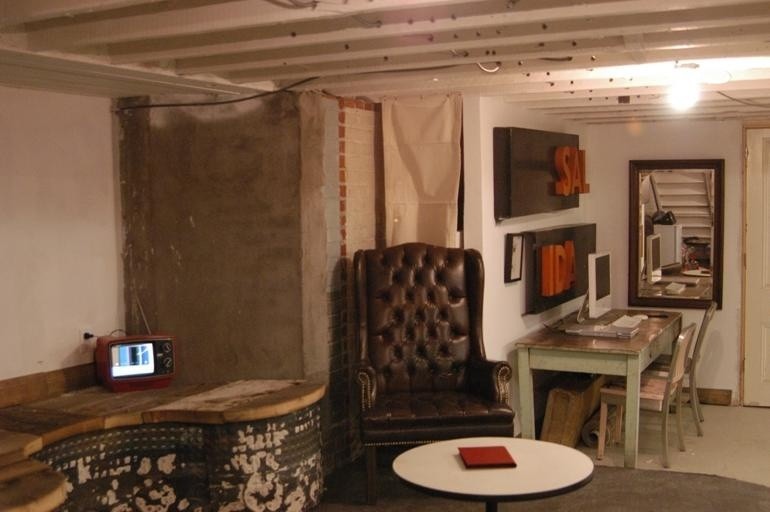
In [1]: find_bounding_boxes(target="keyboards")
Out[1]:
[609,315,642,328]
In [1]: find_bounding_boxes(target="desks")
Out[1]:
[639,262,713,301]
[0,374,326,512]
[516,308,682,468]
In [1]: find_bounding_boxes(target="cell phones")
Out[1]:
[647,313,668,317]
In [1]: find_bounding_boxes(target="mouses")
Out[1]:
[633,314,648,321]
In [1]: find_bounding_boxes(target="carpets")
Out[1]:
[305,467,770,512]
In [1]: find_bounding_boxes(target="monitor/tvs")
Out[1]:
[95,335,177,393]
[576,252,612,326]
[640,233,662,293]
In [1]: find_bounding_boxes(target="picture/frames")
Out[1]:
[504,233,524,282]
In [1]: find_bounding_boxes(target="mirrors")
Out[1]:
[627,159,725,310]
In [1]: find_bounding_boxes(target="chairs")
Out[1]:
[352,243,513,506]
[596,322,697,467]
[642,301,717,438]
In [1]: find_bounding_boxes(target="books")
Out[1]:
[457,446,517,469]
[611,315,642,329]
